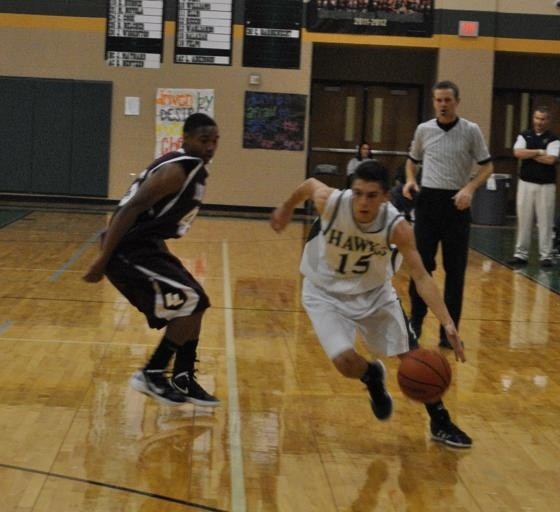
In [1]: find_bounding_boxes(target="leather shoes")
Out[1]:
[507,256,529,267]
[542,259,554,268]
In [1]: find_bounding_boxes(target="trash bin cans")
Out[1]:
[472,172,512,228]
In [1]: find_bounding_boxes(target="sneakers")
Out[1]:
[167,371,222,406]
[407,323,421,349]
[438,335,465,350]
[367,358,394,421]
[128,415,213,464]
[429,410,473,448]
[128,367,187,406]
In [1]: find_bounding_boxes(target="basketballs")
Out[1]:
[397,348,452,404]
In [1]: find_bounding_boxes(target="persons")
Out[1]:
[502,104,559,271]
[345,139,381,177]
[80,112,223,407]
[269,156,475,448]
[400,80,495,349]
